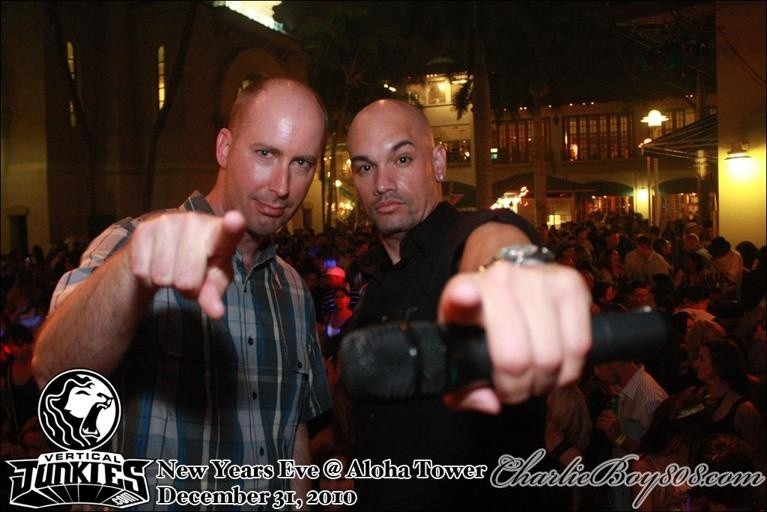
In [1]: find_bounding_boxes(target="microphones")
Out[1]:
[338,317,677,406]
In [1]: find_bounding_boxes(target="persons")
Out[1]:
[333,99,595,511]
[31,80,335,510]
[1,210,766,511]
